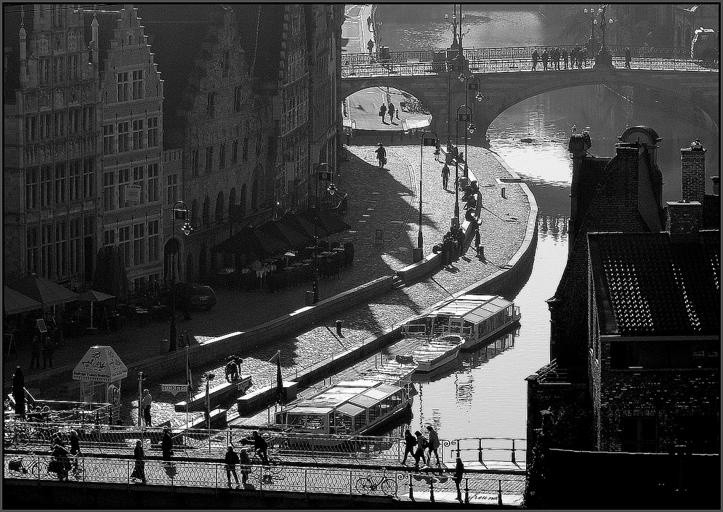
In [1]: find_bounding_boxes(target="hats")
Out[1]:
[241,449,247,452]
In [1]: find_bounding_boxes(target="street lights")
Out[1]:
[367,14,382,62]
[311,162,338,303]
[445,12,471,58]
[583,8,614,53]
[417,59,483,248]
[170,199,195,351]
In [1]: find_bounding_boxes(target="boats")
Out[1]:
[273,293,523,450]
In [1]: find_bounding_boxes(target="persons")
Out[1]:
[367,39,374,55]
[439,227,465,269]
[540,44,587,71]
[441,164,450,190]
[426,425,440,465]
[141,388,153,426]
[41,336,55,370]
[452,457,464,501]
[624,48,631,69]
[56,431,64,447]
[238,448,251,484]
[473,228,482,257]
[530,49,540,72]
[49,444,72,480]
[379,103,386,123]
[413,431,427,465]
[50,433,61,450]
[374,142,386,169]
[68,430,81,465]
[129,440,149,481]
[161,428,171,464]
[366,16,371,31]
[387,103,395,124]
[400,429,415,464]
[252,431,270,470]
[445,139,465,165]
[223,446,240,487]
[27,404,51,423]
[461,180,483,231]
[433,139,442,161]
[28,333,42,371]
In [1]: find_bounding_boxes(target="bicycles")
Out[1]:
[242,445,283,475]
[3,419,88,481]
[356,467,398,497]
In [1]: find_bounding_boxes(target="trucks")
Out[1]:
[690,27,720,69]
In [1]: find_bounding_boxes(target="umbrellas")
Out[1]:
[215,207,351,264]
[77,290,116,327]
[3,284,43,318]
[7,272,80,310]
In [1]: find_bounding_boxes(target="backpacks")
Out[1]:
[419,436,428,449]
